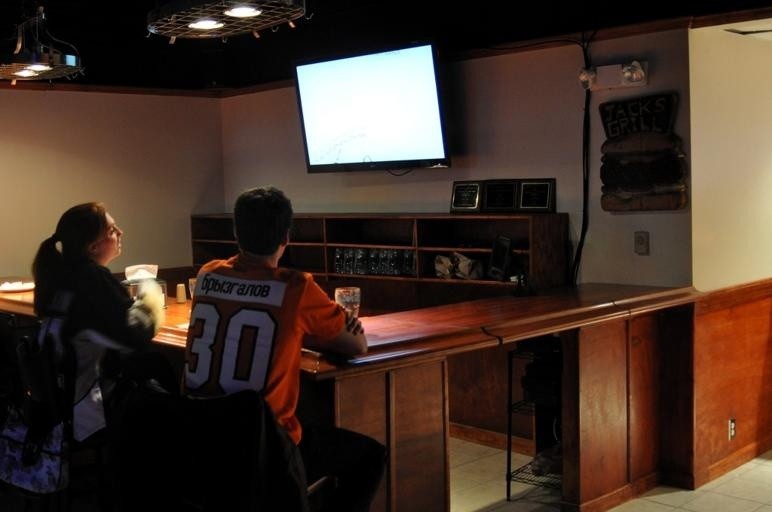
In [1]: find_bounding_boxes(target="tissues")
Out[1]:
[120,264,168,310]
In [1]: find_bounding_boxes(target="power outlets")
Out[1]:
[727,419,737,439]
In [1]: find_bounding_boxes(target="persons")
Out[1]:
[31,201,181,434]
[183,186,388,509]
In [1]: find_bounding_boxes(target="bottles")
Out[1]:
[176,283,187,303]
[330,248,417,278]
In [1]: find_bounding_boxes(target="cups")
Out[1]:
[335,285,361,321]
[188,278,198,300]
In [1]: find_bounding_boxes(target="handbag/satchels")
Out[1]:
[434,252,485,280]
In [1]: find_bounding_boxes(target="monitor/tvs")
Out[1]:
[292,40,452,175]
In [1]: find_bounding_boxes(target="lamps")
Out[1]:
[147,0,306,39]
[579,60,648,90]
[0,0,83,81]
